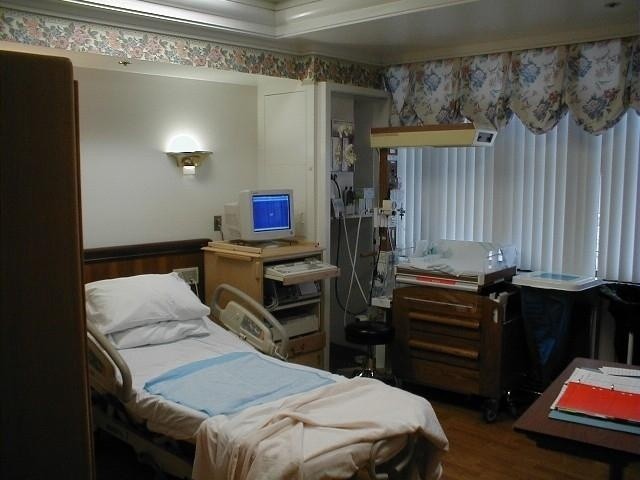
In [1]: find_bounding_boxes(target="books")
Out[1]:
[556,379,639,425]
[547,366,640,426]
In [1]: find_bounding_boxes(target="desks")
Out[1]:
[512,356,639,478]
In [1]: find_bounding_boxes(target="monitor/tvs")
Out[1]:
[225,188,296,242]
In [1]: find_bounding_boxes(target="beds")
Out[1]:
[85,272,428,480]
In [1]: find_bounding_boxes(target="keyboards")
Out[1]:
[266,261,324,278]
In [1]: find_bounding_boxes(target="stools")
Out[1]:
[343,320,397,387]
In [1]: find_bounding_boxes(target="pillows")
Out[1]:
[82,272,213,348]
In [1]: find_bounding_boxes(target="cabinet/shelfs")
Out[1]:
[389,286,521,422]
[199,246,341,367]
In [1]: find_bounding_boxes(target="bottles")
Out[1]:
[342,185,354,206]
